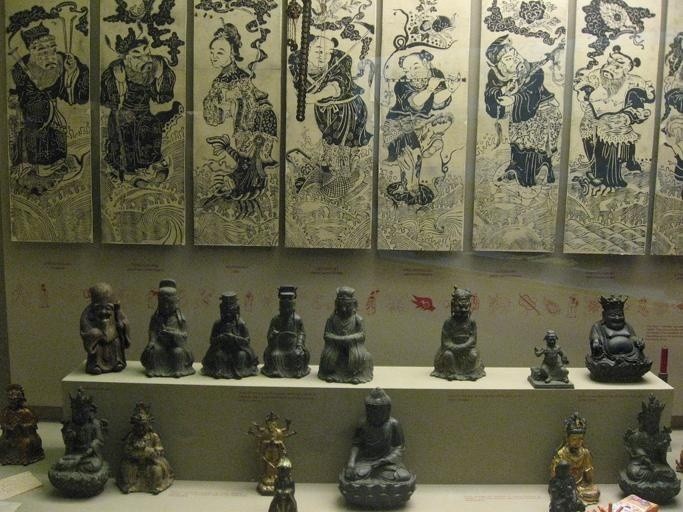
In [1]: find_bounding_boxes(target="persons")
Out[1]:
[116,402,171,495]
[9,23,89,172]
[432,285,476,380]
[201,290,255,379]
[268,451,297,512]
[287,33,373,169]
[383,48,461,198]
[342,387,410,484]
[573,45,653,195]
[51,386,104,473]
[261,285,311,379]
[101,30,176,187]
[79,281,131,375]
[546,460,584,512]
[588,293,647,365]
[139,278,195,378]
[202,23,276,219]
[549,412,599,506]
[247,412,296,480]
[534,330,568,384]
[0,383,41,466]
[482,32,563,191]
[316,286,373,384]
[623,391,675,482]
